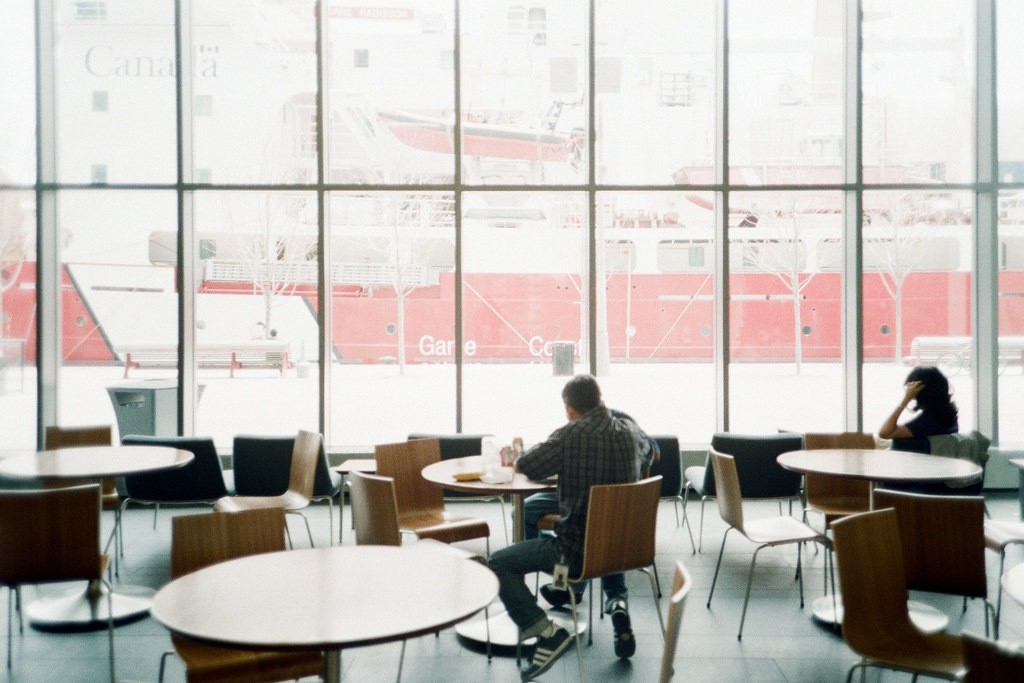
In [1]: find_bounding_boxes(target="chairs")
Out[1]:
[103,430,341,558]
[646,433,876,640]
[0,484,116,683]
[829,488,1024,683]
[350,434,509,559]
[536,477,693,683]
[157,507,323,683]
[896,436,984,496]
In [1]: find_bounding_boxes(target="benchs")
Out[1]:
[115,347,289,378]
[902,337,1024,364]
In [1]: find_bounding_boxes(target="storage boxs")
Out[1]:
[109,382,179,439]
[553,342,575,377]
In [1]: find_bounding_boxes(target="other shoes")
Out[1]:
[540,583,582,608]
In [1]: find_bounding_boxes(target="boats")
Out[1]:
[1,95,1021,364]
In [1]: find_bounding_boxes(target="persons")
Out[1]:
[485,374,660,682]
[877,366,961,455]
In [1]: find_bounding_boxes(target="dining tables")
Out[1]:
[0,446,195,632]
[149,542,499,683]
[776,449,982,634]
[420,455,585,647]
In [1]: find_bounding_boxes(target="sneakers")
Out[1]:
[521,623,574,682]
[611,600,636,657]
